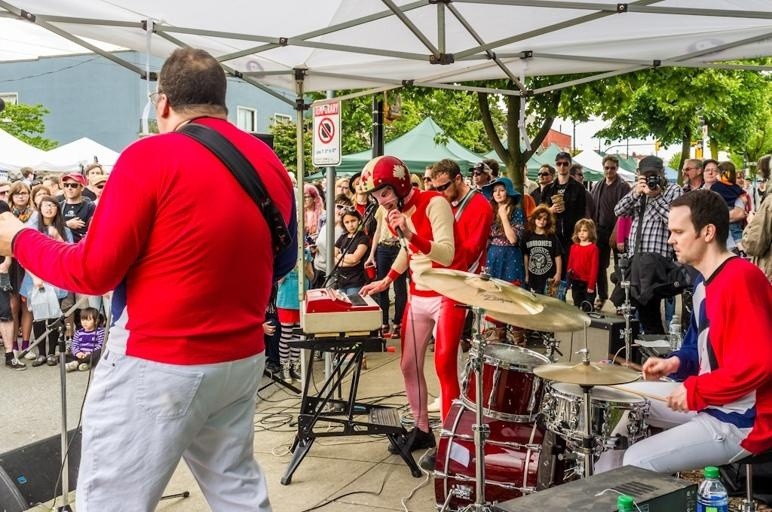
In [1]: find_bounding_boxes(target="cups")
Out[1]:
[366,265,377,281]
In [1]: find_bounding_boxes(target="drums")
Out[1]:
[459,340,551,423]
[470,329,554,362]
[542,381,651,453]
[434,398,557,511]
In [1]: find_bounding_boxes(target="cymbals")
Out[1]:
[533,360,641,385]
[422,269,544,316]
[484,291,591,333]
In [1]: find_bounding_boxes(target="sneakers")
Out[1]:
[386,424,436,452]
[76,361,89,372]
[294,364,301,381]
[281,365,292,384]
[30,353,46,367]
[22,346,35,360]
[46,352,59,365]
[63,358,78,371]
[3,354,27,370]
[11,348,19,359]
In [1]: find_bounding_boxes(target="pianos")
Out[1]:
[298,286,383,338]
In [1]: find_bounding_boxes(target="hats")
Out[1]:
[480,176,521,205]
[637,154,664,177]
[349,173,361,192]
[60,171,88,188]
[469,161,492,180]
[90,175,110,186]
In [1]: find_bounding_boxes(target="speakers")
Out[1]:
[0,422,83,512]
[554,314,640,369]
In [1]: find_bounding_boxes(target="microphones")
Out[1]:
[387,208,408,253]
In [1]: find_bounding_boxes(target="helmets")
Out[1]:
[357,155,413,200]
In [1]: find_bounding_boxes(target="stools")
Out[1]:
[734,449,772,512]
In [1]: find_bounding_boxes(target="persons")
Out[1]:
[358,157,469,454]
[592,188,772,477]
[0,150,771,414]
[0,48,294,512]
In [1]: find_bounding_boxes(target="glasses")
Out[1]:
[61,183,78,189]
[573,172,584,176]
[304,195,312,200]
[680,167,692,172]
[147,90,160,104]
[0,189,10,196]
[535,172,549,177]
[431,182,452,191]
[555,160,568,167]
[13,192,27,198]
[96,185,104,189]
[423,176,431,181]
[335,202,357,212]
[469,170,480,176]
[603,165,615,170]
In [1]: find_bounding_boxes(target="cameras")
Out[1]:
[645,174,668,190]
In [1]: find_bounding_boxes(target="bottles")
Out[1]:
[696,466,729,512]
[669,315,682,351]
[616,495,634,512]
